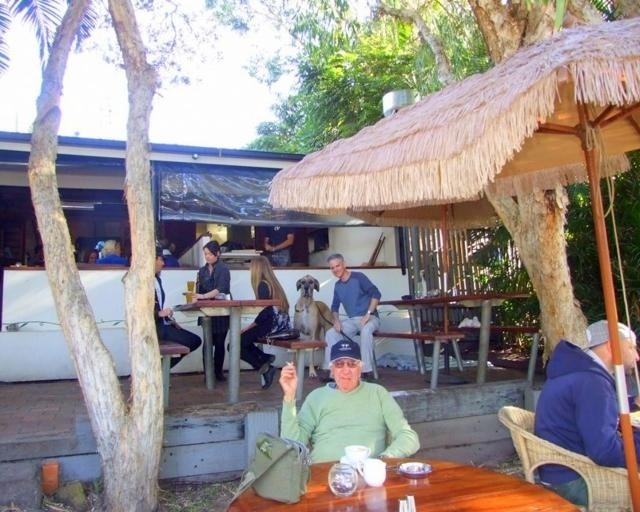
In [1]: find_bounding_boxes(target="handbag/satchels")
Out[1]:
[225,432,312,509]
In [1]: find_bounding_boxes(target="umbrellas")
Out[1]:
[266,17,640,511]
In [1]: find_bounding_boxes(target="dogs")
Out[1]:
[292,275,337,379]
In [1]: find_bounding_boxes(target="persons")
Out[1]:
[95,239,129,267]
[227,255,290,390]
[152,246,202,370]
[263,209,295,267]
[321,254,382,382]
[192,240,231,383]
[534,320,640,506]
[277,339,420,461]
[83,248,99,264]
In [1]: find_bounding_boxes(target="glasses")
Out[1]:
[334,361,355,368]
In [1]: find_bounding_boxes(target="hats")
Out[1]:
[584,320,637,348]
[330,340,362,362]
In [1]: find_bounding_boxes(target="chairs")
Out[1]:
[498,404,640,512]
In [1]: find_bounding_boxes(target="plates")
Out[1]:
[399,462,432,477]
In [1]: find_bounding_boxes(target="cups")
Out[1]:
[359,460,387,487]
[344,445,370,465]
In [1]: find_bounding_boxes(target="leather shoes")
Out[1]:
[263,353,276,365]
[261,365,276,390]
[214,370,226,381]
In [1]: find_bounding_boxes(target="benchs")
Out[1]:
[448,325,541,384]
[256,333,328,401]
[158,337,190,407]
[357,327,465,388]
